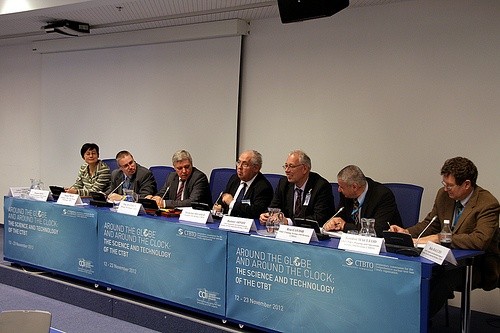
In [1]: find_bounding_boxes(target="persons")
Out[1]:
[209,150,273,220]
[144,149,212,209]
[259,149,336,227]
[387,155,499,318]
[323,166,406,239]
[65,143,111,196]
[107,151,156,201]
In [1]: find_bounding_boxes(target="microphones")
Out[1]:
[294,206,344,241]
[89,181,125,207]
[49,177,79,200]
[191,191,223,218]
[382,215,438,255]
[137,186,170,216]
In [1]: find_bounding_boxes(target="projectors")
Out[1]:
[39,20,90,38]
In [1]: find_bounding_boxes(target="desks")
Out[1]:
[4,195,486,333]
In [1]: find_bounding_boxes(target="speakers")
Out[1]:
[276,0,350,24]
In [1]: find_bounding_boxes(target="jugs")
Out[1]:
[265,207,284,237]
[359,217,376,238]
[125,189,138,203]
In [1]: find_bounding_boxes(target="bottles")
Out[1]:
[439,219,453,250]
[29,182,40,189]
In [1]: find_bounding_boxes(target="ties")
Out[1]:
[177,179,185,200]
[452,200,464,228]
[237,183,248,202]
[294,187,304,217]
[351,198,362,226]
[122,176,132,196]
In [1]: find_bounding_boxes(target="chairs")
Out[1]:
[382,183,424,228]
[0,310,52,333]
[209,168,236,205]
[148,166,177,191]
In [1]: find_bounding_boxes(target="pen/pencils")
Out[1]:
[335,222,340,225]
[387,222,391,227]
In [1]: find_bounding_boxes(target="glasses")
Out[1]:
[441,180,457,189]
[283,165,302,169]
[236,160,253,169]
